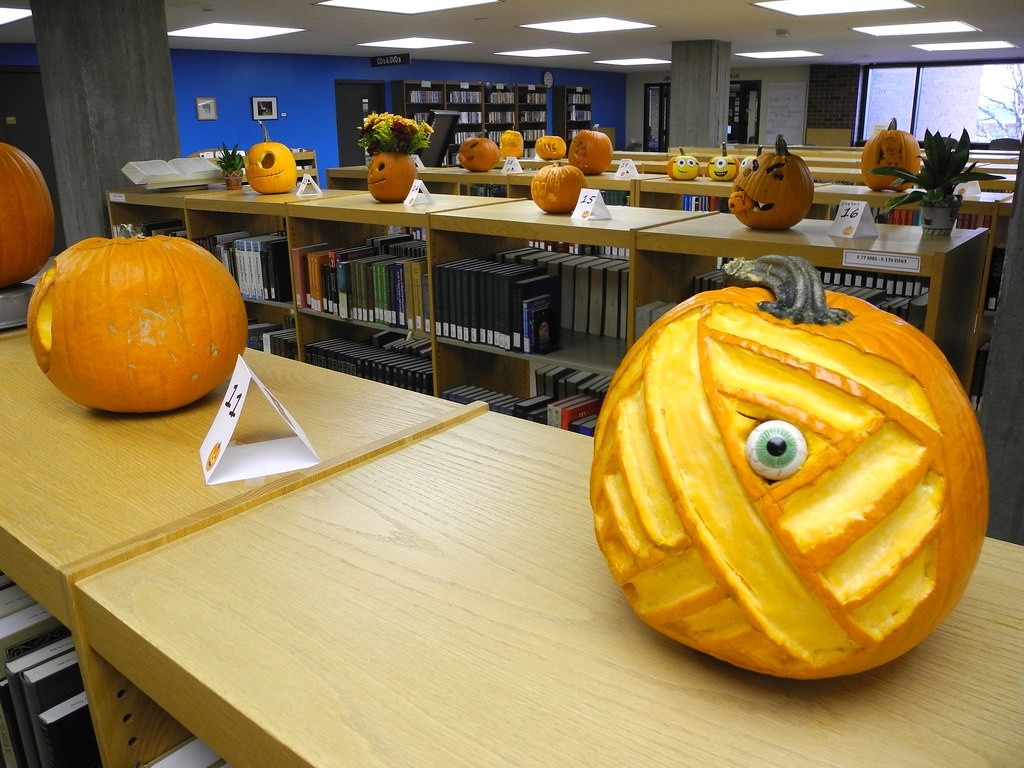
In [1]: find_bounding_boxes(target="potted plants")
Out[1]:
[865,127,1006,235]
[213,142,245,189]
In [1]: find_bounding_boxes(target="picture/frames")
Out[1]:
[252,96,278,120]
[196,97,217,121]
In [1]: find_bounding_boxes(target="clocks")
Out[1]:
[543,70,554,88]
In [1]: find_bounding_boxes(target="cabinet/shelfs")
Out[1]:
[0,0,1024,768]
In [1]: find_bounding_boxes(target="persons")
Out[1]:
[40,144,66,244]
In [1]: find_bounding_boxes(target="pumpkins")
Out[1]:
[0,141,55,289]
[532,161,588,214]
[666,147,701,180]
[708,142,740,181]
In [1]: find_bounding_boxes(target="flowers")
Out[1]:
[356,112,434,154]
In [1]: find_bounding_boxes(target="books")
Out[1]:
[110,167,1013,437]
[0,570,232,768]
[410,91,591,198]
